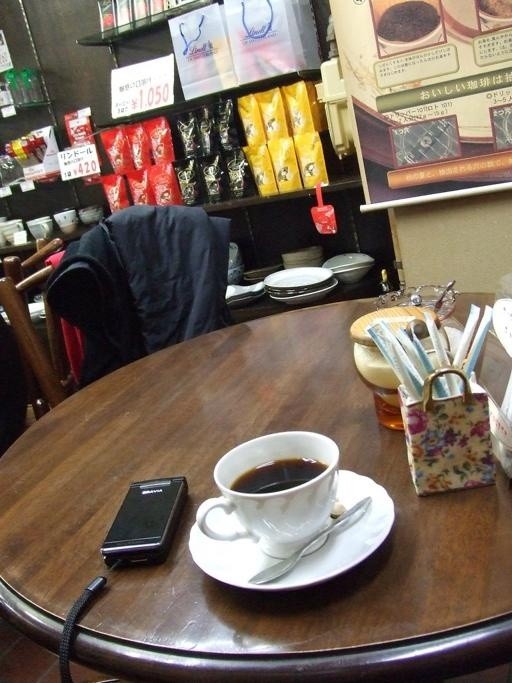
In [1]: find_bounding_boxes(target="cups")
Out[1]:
[197,431,341,558]
[350,307,452,432]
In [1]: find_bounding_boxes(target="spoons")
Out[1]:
[249,497,371,584]
[434,279,456,314]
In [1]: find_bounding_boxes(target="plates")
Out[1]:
[0,204,102,248]
[0,294,46,326]
[378,10,512,47]
[189,470,395,592]
[226,243,375,308]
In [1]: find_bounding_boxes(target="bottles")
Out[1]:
[0,67,44,108]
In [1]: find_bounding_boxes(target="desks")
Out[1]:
[0,293,512,683]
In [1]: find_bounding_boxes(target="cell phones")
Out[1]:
[100,475,188,569]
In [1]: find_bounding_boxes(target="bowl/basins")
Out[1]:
[377,284,460,321]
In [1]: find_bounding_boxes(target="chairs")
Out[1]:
[1,239,67,418]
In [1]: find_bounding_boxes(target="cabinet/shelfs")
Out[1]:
[77,0,402,321]
